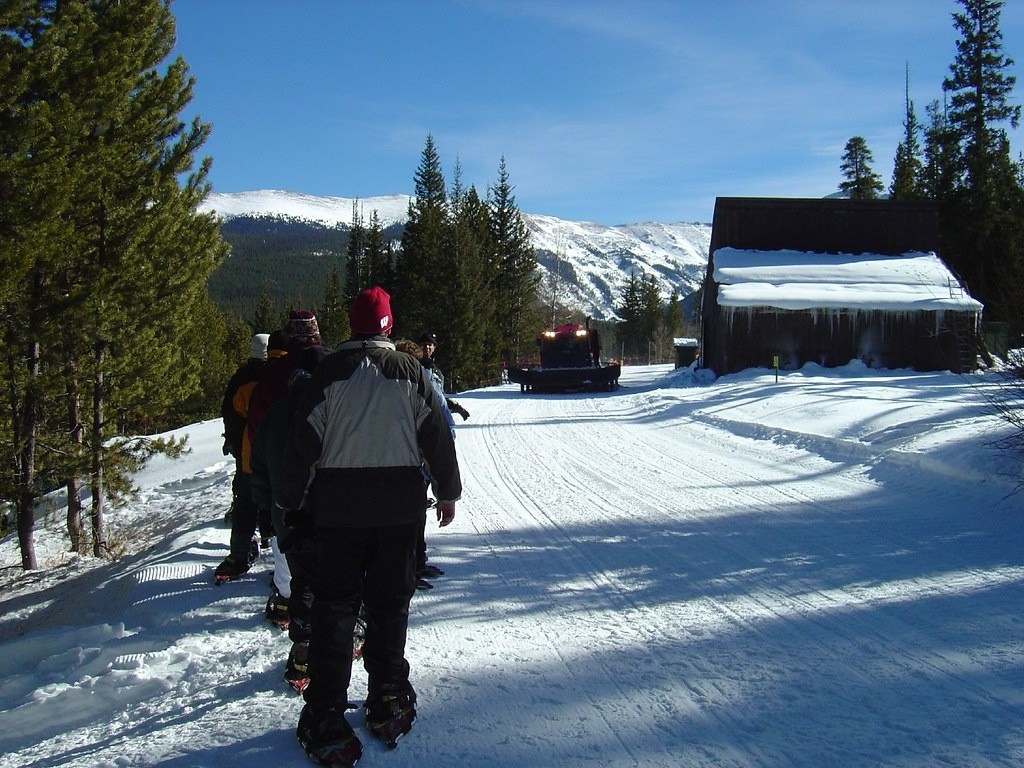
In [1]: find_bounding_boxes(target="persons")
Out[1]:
[243,309,346,621]
[271,354,374,690]
[214,309,473,589]
[275,287,462,761]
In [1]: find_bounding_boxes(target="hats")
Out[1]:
[419,331,438,346]
[251,333,271,358]
[350,286,394,334]
[287,310,321,348]
[267,329,289,353]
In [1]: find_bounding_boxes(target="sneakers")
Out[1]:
[297,705,364,768]
[215,553,250,586]
[284,643,312,694]
[352,618,368,661]
[416,579,433,589]
[365,692,417,749]
[424,565,445,578]
[265,593,292,634]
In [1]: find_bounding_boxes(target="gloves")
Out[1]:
[457,405,470,421]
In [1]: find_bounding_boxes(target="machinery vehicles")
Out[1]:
[507,315,622,396]
